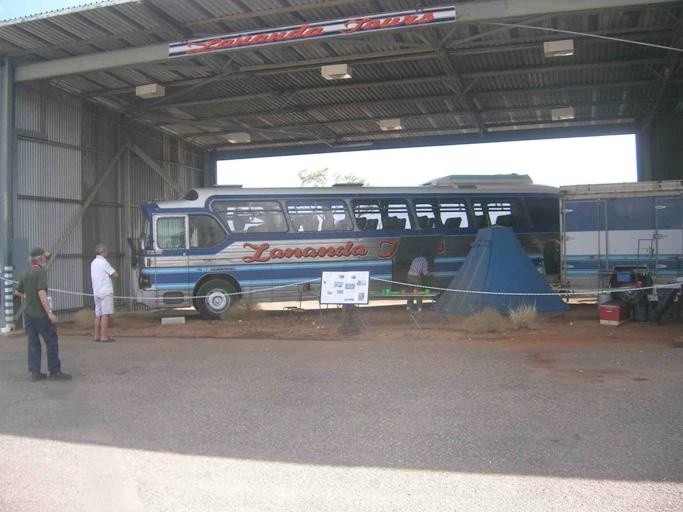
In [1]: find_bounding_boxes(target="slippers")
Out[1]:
[96,337,115,342]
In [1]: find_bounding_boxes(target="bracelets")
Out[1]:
[46,309,52,313]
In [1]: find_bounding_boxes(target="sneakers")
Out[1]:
[32,373,47,380]
[49,370,72,380]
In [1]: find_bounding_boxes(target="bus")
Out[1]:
[128,171,563,320]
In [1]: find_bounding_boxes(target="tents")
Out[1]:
[426,223,577,316]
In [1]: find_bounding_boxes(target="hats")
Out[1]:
[31,247,50,257]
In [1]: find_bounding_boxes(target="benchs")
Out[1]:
[367,291,441,311]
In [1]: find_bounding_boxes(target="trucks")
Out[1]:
[557,177,683,306]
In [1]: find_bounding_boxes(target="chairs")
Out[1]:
[356,215,514,233]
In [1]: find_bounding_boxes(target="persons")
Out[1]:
[404,251,432,311]
[14,247,72,380]
[88,242,117,342]
